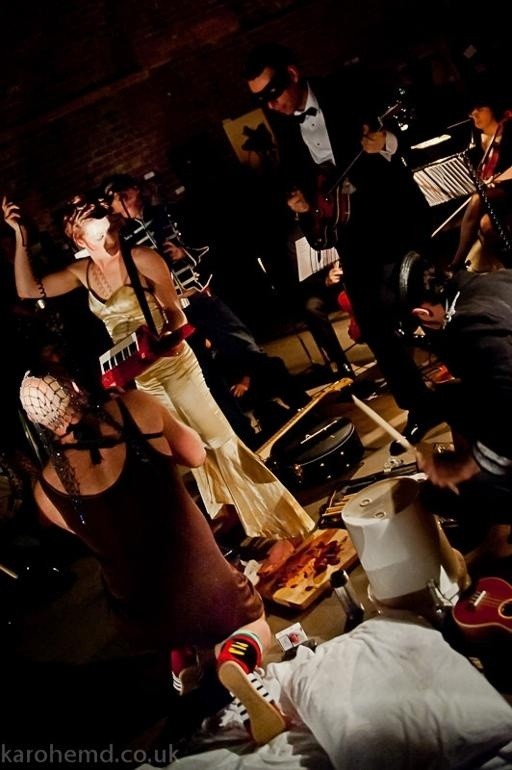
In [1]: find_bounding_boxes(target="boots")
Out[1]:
[215,631,288,749]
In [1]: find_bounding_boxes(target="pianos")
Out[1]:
[98,324,140,377]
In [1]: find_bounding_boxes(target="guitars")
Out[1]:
[453,577,512,640]
[299,87,413,249]
[253,378,354,463]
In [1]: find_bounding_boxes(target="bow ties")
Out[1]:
[296,107,318,123]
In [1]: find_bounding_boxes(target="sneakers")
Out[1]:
[169,647,201,697]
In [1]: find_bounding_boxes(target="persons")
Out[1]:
[1,0,511,750]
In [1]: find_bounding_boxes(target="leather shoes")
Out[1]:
[389,422,424,456]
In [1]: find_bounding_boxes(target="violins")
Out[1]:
[478,109,512,185]
